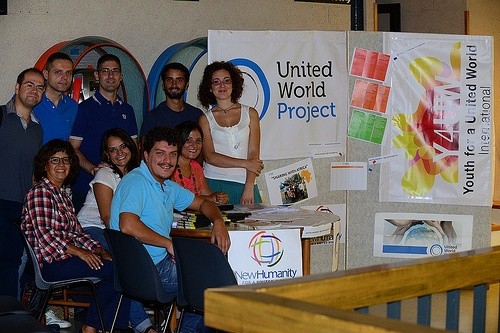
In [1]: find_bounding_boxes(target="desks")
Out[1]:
[169,203,341,333]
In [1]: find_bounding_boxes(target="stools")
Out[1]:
[0,295,58,333]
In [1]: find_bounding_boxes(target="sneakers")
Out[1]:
[41,309,72,328]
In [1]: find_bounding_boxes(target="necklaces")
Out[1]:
[177,164,198,197]
[216,103,235,113]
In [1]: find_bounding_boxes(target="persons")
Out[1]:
[21,139,131,333]
[171,120,230,205]
[69,55,138,213]
[109,127,231,333]
[197,61,264,204]
[141,63,203,146]
[32,52,79,143]
[282,182,305,202]
[0,68,74,329]
[78,129,159,333]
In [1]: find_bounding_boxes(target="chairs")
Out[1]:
[21,230,107,333]
[102,228,178,333]
[172,237,239,333]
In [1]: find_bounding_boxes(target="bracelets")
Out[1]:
[98,248,104,255]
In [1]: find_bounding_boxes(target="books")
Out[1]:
[222,213,251,224]
[172,211,210,230]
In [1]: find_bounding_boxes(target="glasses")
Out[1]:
[22,82,45,92]
[97,68,120,74]
[211,79,233,85]
[49,157,72,165]
